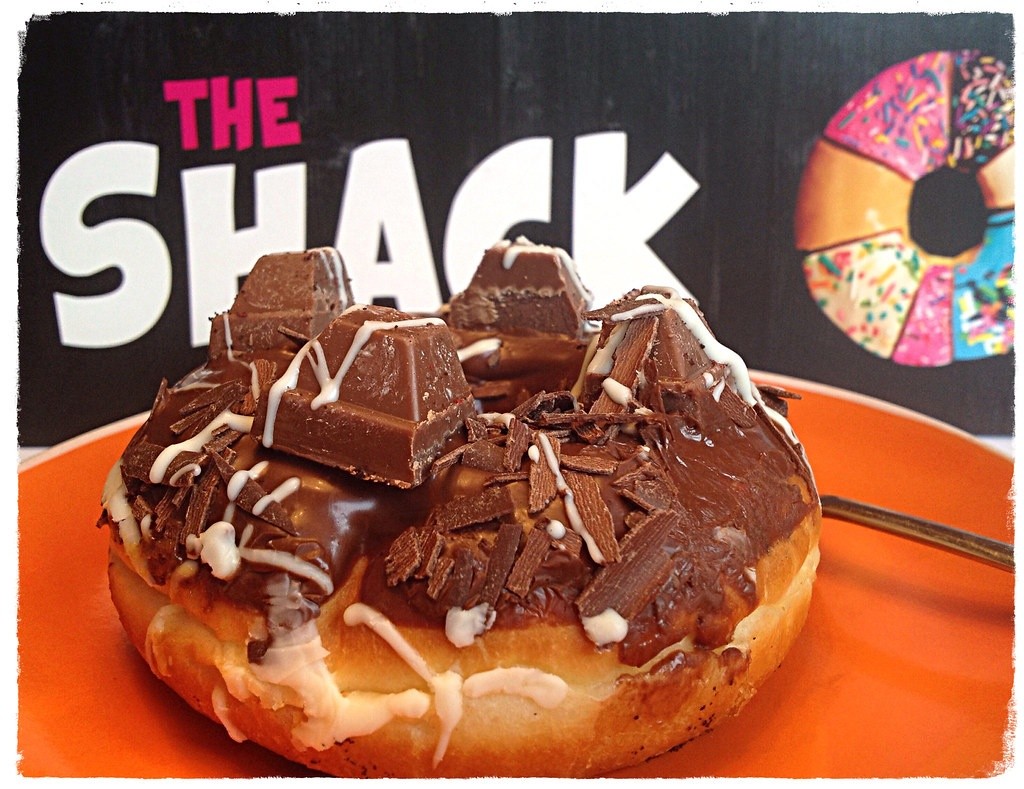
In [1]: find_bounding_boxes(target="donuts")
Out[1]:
[795,48,1016,366]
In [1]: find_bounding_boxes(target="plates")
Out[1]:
[19,367,1013,779]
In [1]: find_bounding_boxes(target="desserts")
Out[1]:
[94,236,825,778]
